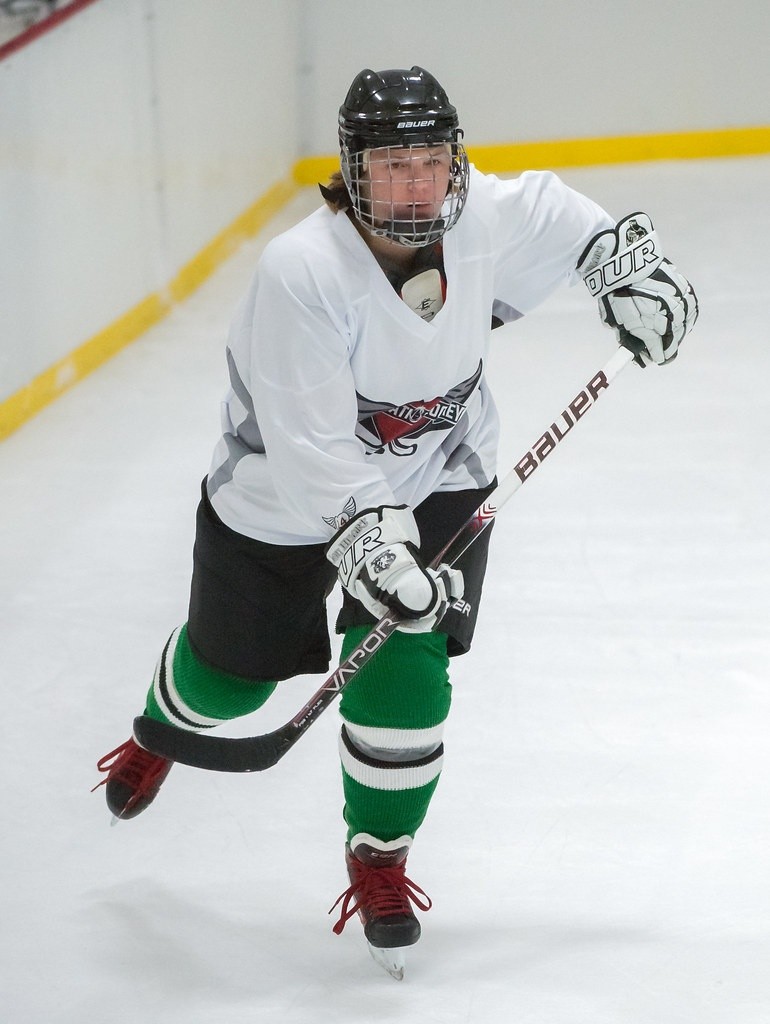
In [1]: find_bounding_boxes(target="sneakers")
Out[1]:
[328,831,432,982]
[89,735,176,828]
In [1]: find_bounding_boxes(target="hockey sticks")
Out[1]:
[132,343,634,773]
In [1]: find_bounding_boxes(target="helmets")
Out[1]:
[337,65,461,193]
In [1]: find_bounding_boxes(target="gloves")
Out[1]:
[323,503,465,634]
[572,210,700,371]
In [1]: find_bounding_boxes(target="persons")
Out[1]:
[99,64,702,959]
